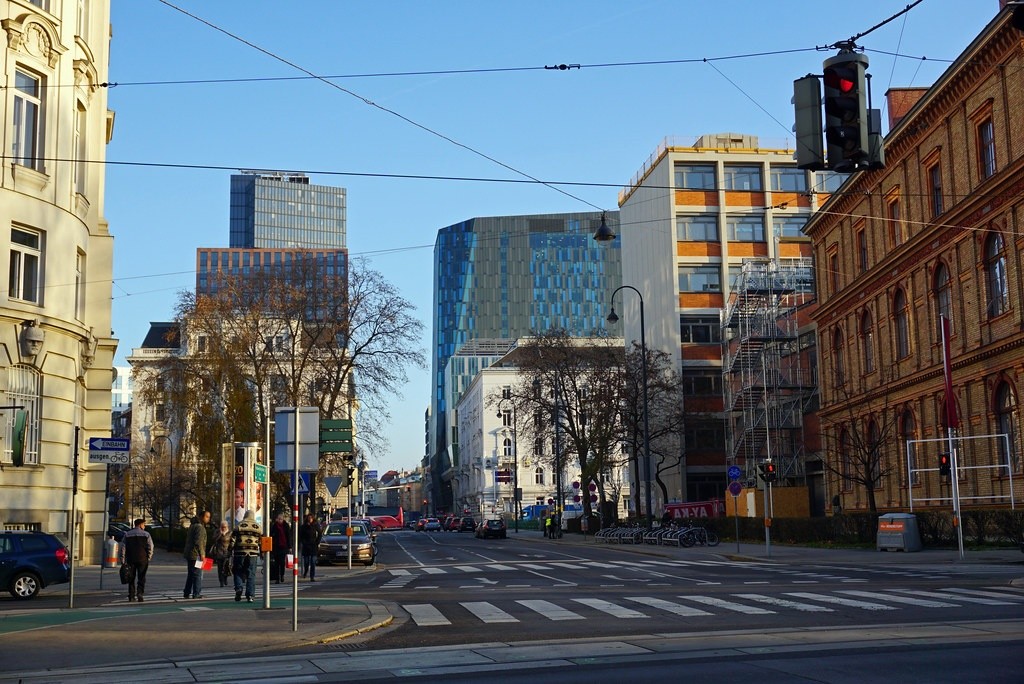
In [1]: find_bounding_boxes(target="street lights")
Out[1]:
[148,434,174,554]
[607,285,654,535]
[495,398,518,535]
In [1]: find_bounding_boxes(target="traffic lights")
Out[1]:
[822,61,867,171]
[758,465,767,482]
[342,467,356,488]
[939,453,949,476]
[764,463,776,483]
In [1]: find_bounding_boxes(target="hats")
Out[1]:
[134,519,146,525]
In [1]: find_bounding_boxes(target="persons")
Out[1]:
[299,513,322,582]
[225,487,244,530]
[213,519,234,587]
[333,510,343,520]
[183,511,211,599]
[546,514,556,539]
[270,513,292,584]
[228,510,265,602]
[120,518,154,602]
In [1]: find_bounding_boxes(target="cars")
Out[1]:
[442,516,453,532]
[473,522,484,538]
[315,517,380,566]
[424,518,441,532]
[405,520,426,532]
[448,518,462,532]
[457,517,476,533]
[480,520,507,539]
[0,529,72,602]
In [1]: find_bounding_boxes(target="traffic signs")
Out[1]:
[88,436,129,453]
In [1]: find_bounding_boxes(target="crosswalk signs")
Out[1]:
[290,472,313,495]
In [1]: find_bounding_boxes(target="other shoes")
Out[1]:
[220,582,225,587]
[303,570,308,578]
[138,598,143,602]
[311,578,315,582]
[224,578,228,585]
[234,589,242,601]
[193,595,204,599]
[275,581,279,584]
[130,598,136,602]
[248,596,254,602]
[281,575,284,582]
[184,592,191,599]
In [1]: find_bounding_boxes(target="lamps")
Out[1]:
[593,209,616,246]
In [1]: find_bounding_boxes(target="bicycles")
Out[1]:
[600,518,720,548]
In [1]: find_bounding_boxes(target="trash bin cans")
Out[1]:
[876,513,922,553]
[581,517,588,531]
[104,538,119,569]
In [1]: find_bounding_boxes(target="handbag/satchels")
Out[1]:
[285,551,293,569]
[120,563,133,585]
